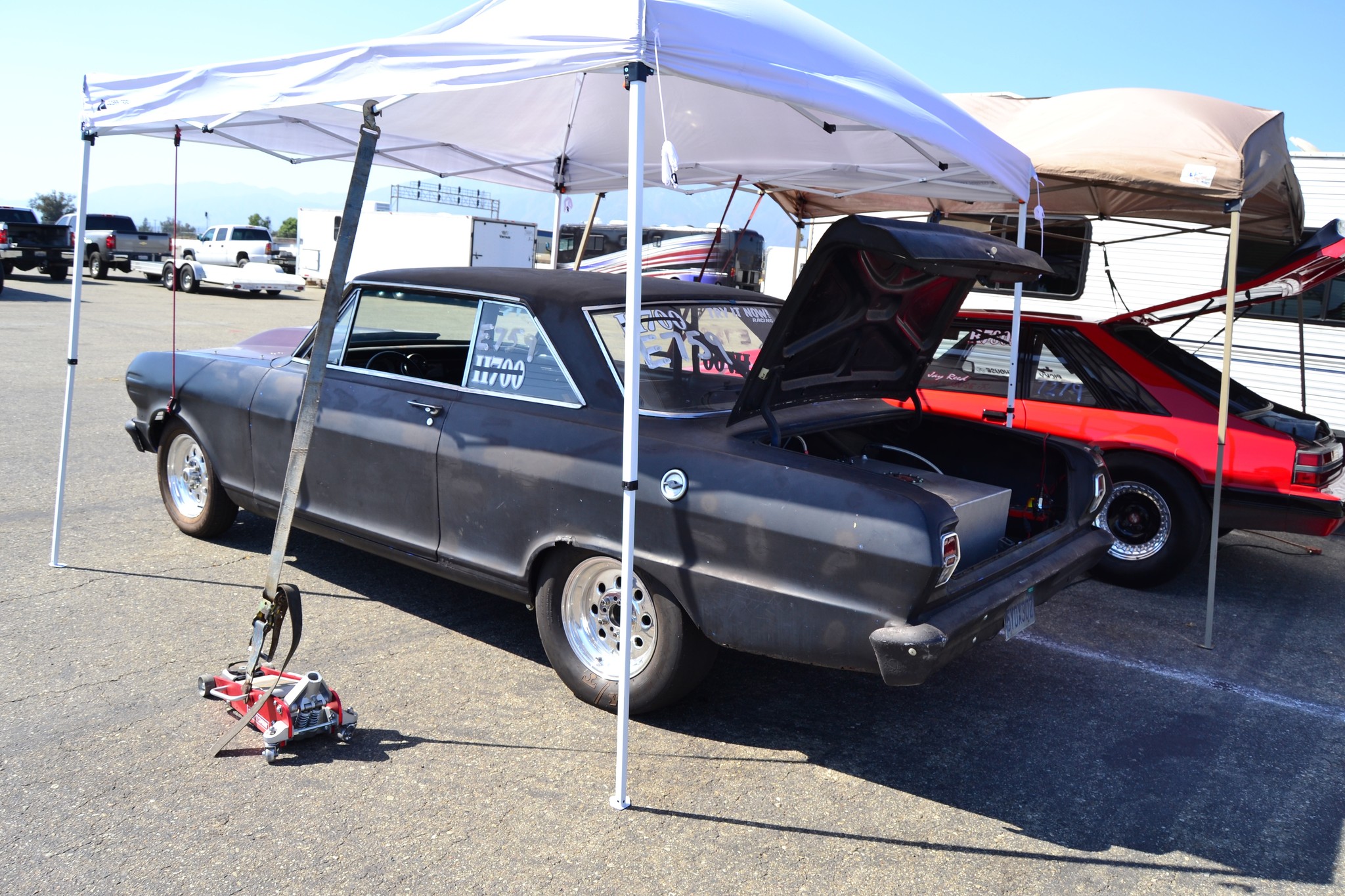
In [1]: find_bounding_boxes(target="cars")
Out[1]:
[122,213,1115,719]
[690,217,1345,591]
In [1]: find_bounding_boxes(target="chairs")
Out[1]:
[623,367,695,414]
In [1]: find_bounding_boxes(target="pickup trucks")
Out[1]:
[0,205,72,281]
[53,213,173,280]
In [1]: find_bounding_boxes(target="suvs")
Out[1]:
[179,225,279,268]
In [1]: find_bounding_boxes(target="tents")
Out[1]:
[50,0,1037,813]
[574,88,1307,652]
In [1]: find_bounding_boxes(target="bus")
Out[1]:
[546,218,767,295]
